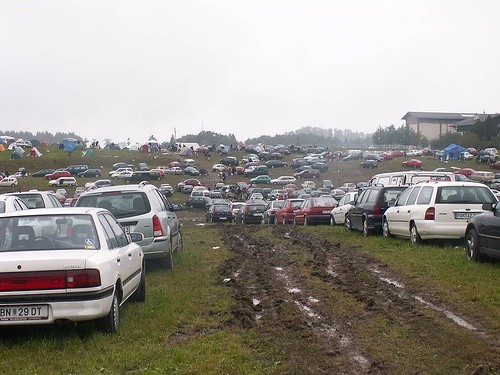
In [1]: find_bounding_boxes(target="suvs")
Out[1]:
[110,162,163,184]
[32,164,101,186]
[14,140,32,148]
[0,180,184,334]
[343,180,499,245]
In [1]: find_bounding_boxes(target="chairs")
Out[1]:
[12,226,35,246]
[134,197,145,210]
[72,223,92,245]
[36,201,44,207]
[98,201,112,210]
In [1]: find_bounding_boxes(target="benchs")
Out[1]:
[448,193,475,201]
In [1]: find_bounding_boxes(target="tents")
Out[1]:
[0,136,230,160]
[444,144,467,160]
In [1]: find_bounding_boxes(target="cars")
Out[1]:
[156,161,200,176]
[242,144,305,168]
[289,146,330,179]
[0,171,28,187]
[360,149,424,168]
[458,148,500,169]
[464,201,500,262]
[456,168,500,189]
[177,178,367,232]
[211,163,227,172]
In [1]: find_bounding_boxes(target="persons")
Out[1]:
[22,168,26,178]
[68,150,237,184]
[7,171,10,177]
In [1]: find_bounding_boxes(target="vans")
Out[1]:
[368,171,455,187]
[182,158,197,168]
[243,165,268,175]
[220,156,239,166]
